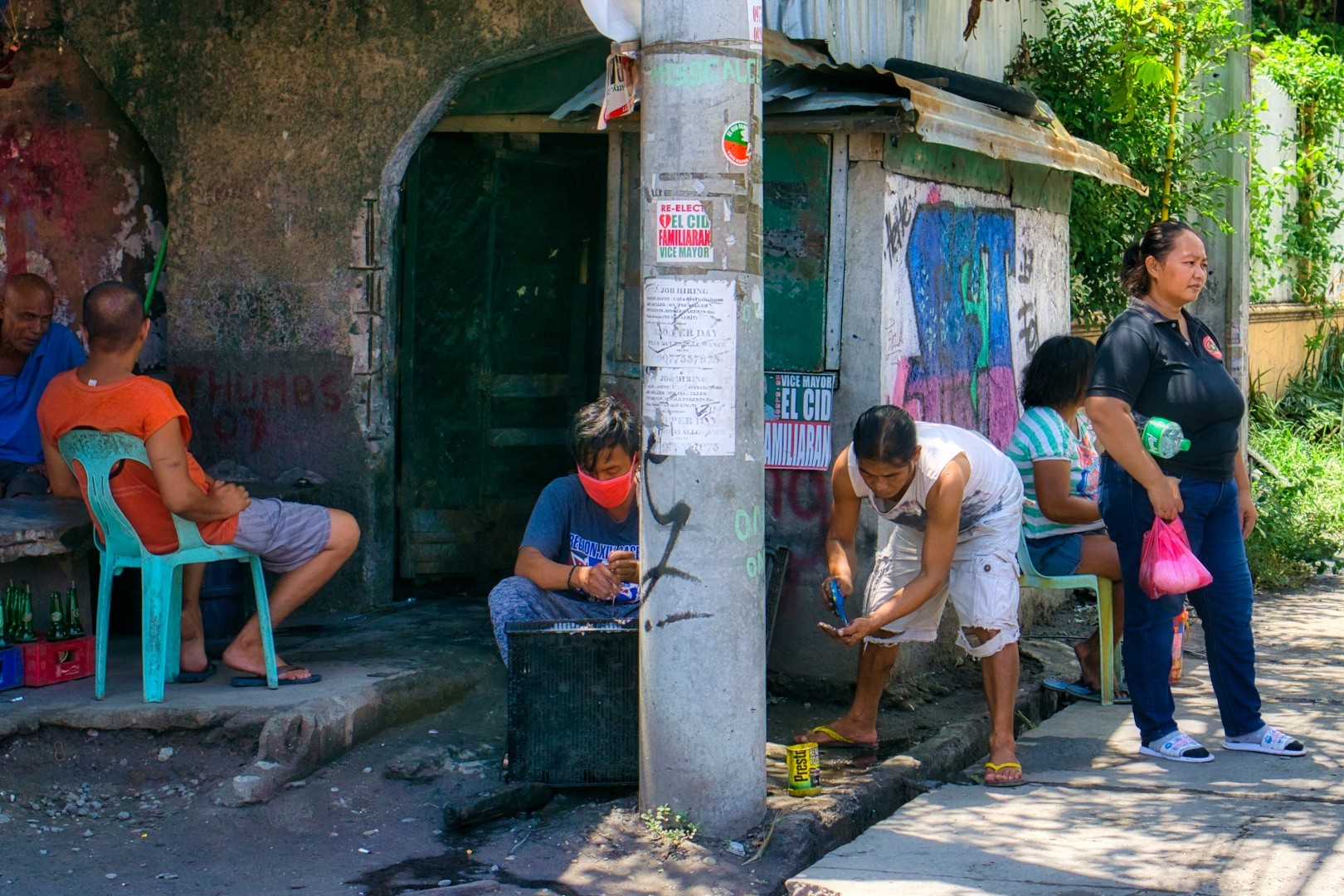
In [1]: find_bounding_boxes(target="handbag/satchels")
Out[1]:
[1140,512,1214,600]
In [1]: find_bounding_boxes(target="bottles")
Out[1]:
[0,584,87,664]
[1169,605,1188,683]
[785,742,822,797]
[1087,408,1192,461]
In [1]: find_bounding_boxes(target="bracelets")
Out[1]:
[567,565,583,593]
[488,395,642,769]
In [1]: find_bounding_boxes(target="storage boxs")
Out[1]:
[0,635,96,693]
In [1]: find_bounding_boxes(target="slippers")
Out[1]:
[797,725,879,751]
[1042,677,1087,692]
[231,665,323,687]
[1138,732,1217,763]
[1066,679,1133,704]
[1223,727,1308,756]
[177,659,218,684]
[984,759,1026,787]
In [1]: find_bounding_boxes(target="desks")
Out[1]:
[0,493,94,635]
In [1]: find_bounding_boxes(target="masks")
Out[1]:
[576,450,637,509]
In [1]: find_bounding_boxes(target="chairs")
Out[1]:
[51,424,284,704]
[1012,521,1123,711]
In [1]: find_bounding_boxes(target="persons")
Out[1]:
[37,279,361,688]
[794,404,1024,787]
[1006,336,1133,706]
[0,273,94,636]
[1085,219,1308,763]
